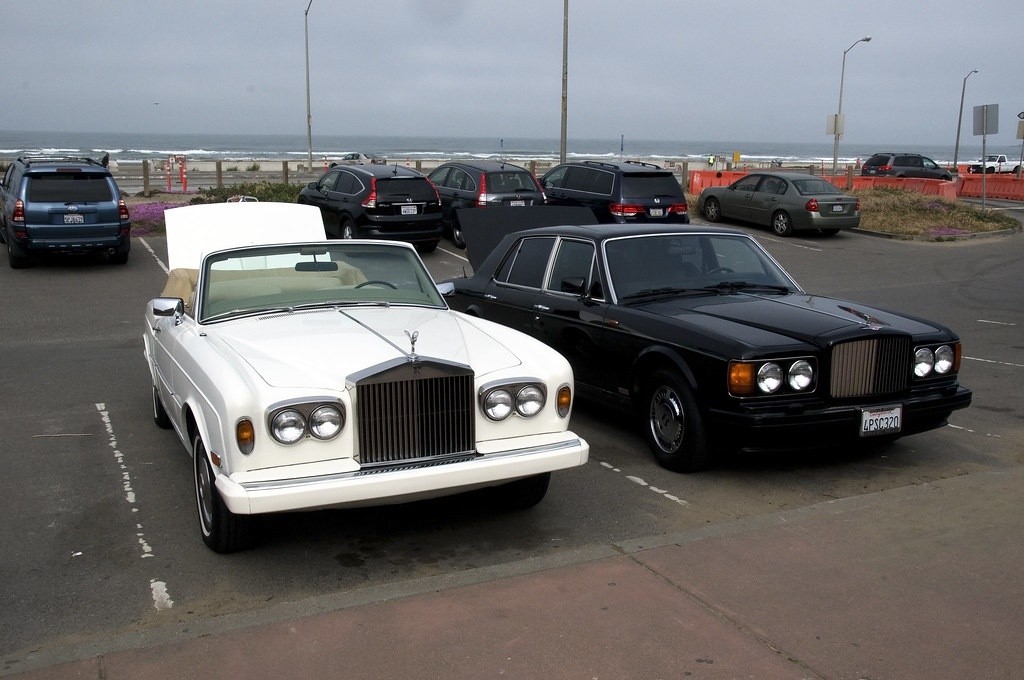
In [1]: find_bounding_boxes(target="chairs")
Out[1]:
[189,282,248,319]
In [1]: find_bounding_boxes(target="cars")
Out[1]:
[696,173,862,237]
[434,203,974,474]
[142,202,592,555]
[329,153,386,168]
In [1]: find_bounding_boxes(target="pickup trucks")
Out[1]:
[967,154,1024,174]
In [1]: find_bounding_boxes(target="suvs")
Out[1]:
[861,152,953,181]
[0,156,131,270]
[296,163,443,254]
[539,159,691,226]
[426,159,549,250]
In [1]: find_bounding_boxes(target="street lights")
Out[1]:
[952,70,979,173]
[832,36,872,175]
